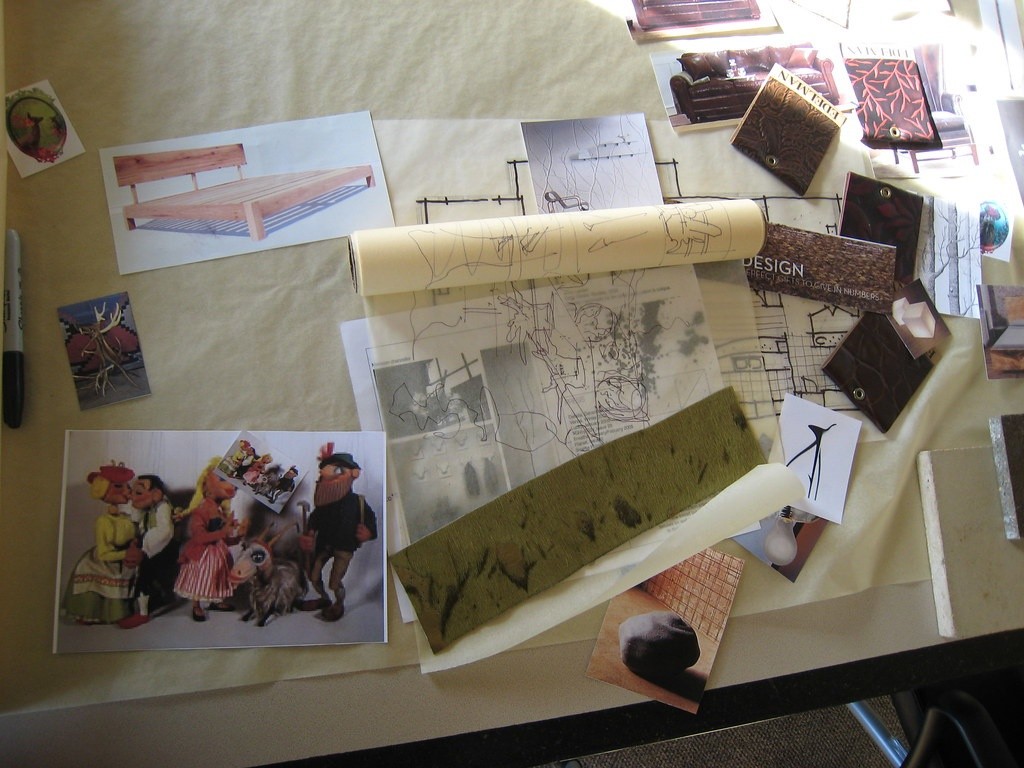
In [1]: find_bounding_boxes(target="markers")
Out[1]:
[2,226,25,434]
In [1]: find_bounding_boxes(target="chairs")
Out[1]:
[845,670,1024,768]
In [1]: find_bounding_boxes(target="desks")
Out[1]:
[0,0,1024,768]
